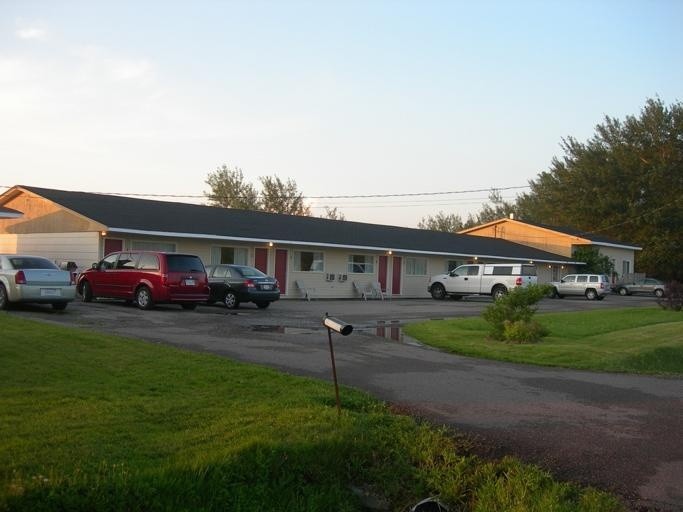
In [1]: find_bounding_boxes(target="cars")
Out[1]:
[616,278,666,298]
[0,254,77,310]
[205,264,280,309]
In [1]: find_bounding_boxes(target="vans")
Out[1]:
[76,251,211,310]
[427,264,537,301]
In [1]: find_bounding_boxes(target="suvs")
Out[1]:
[546,274,612,300]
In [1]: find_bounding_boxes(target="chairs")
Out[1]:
[353,280,392,302]
[296,279,317,303]
